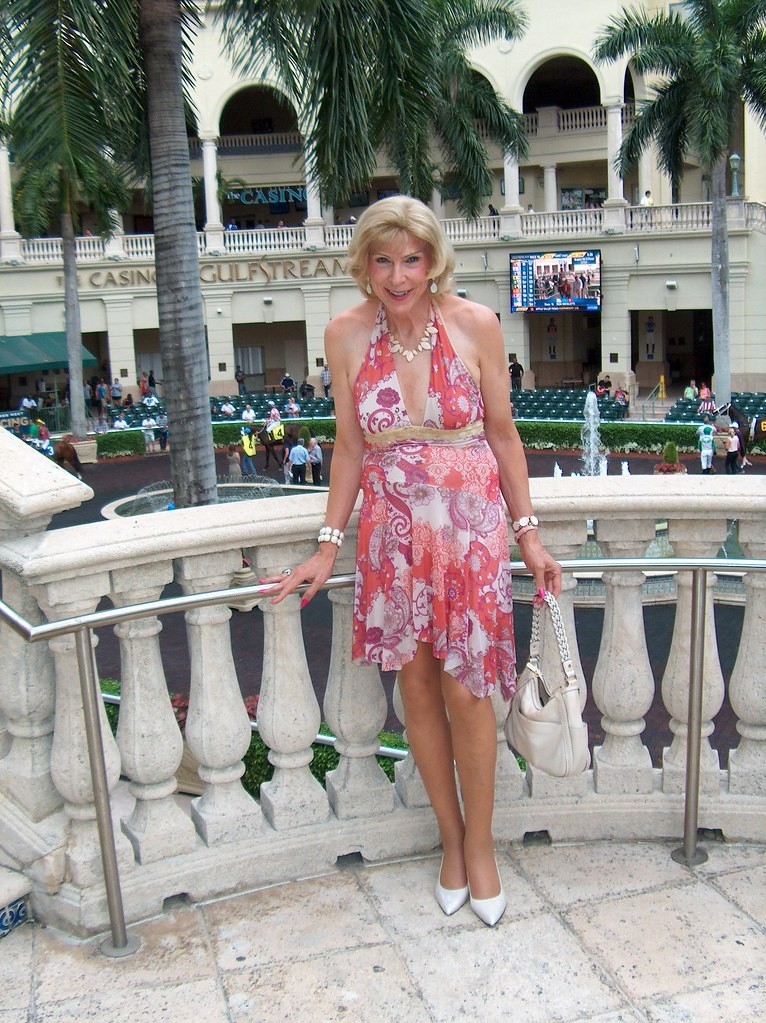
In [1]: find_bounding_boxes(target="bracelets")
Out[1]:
[318,527,345,547]
[512,516,538,542]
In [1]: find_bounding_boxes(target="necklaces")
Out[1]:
[389,300,439,363]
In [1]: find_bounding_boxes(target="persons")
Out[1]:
[213,365,331,486]
[696,417,748,475]
[275,220,287,228]
[226,219,237,230]
[259,196,561,928]
[528,204,536,223]
[85,229,93,236]
[509,358,523,391]
[488,204,500,236]
[684,380,715,419]
[335,215,356,245]
[12,370,168,455]
[255,220,264,228]
[535,271,594,298]
[598,375,629,418]
[641,191,653,206]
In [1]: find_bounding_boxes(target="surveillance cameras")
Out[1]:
[217,308,222,314]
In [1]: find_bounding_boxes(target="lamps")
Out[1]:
[264,297,272,304]
[666,281,677,289]
[458,289,466,297]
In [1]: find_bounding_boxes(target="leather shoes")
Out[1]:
[435,853,468,916]
[468,856,507,927]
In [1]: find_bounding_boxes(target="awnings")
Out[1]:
[0,332,97,431]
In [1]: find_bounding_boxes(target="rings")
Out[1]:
[282,569,292,575]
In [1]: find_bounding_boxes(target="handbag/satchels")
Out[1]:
[504,591,588,778]
[596,387,606,396]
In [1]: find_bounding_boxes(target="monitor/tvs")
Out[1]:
[510,249,602,313]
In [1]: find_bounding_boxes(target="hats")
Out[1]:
[268,400,276,408]
[36,418,46,425]
[729,422,739,428]
[574,275,578,278]
[244,428,251,433]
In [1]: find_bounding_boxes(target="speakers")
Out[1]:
[264,297,273,304]
[666,281,677,289]
[457,289,468,298]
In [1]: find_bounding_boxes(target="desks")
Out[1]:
[265,385,283,393]
[561,378,582,388]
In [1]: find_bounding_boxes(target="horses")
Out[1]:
[243,424,301,472]
[712,403,766,470]
[558,272,572,299]
[48,442,86,479]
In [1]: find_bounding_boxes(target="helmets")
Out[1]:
[704,428,711,435]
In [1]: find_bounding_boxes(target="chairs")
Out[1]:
[210,392,335,421]
[107,399,167,428]
[510,386,629,422]
[664,392,766,423]
